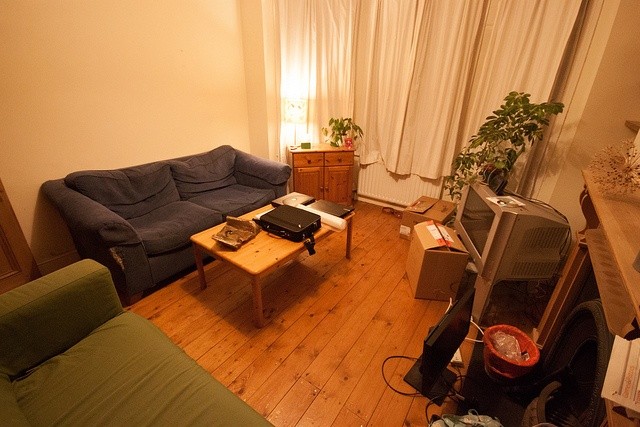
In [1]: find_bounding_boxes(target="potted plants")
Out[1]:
[320,115,365,147]
[441,88,566,204]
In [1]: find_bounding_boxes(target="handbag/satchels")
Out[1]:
[260,205,321,242]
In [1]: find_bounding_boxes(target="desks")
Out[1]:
[187,191,358,327]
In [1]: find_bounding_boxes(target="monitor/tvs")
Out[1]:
[402,287,476,406]
[455,178,569,282]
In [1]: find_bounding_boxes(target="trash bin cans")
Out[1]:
[484,324,541,384]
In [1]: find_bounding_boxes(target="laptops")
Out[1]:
[272,191,315,208]
[305,199,355,218]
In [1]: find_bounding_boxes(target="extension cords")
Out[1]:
[451,348,463,367]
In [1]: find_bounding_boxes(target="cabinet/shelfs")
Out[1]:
[532,164,640,425]
[286,143,354,206]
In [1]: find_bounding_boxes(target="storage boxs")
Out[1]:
[405,219,469,302]
[399,194,458,241]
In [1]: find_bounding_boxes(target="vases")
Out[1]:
[487,149,518,196]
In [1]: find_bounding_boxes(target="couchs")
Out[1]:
[1,258,273,426]
[39,144,292,307]
